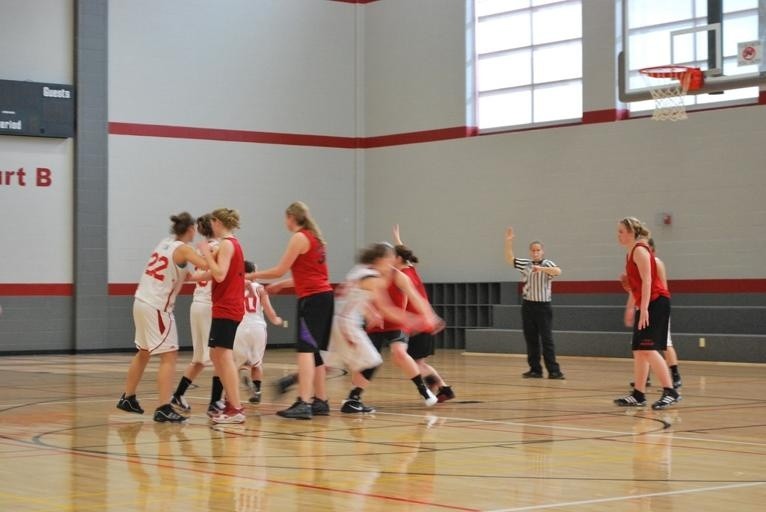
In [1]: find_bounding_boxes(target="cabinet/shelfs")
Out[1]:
[416,282,521,352]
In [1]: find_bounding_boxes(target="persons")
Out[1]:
[612,216,685,410]
[117,210,218,423]
[502,224,567,381]
[171,212,224,414]
[620,239,686,390]
[199,209,249,423]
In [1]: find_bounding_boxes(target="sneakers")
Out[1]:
[614,395,648,406]
[630,380,651,387]
[340,399,376,414]
[521,369,564,380]
[247,391,261,402]
[116,392,144,414]
[275,395,312,420]
[419,383,456,409]
[204,399,248,424]
[170,394,192,412]
[154,409,190,424]
[311,397,330,415]
[652,390,682,408]
[657,379,682,391]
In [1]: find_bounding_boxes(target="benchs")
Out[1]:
[463,295,765,366]
